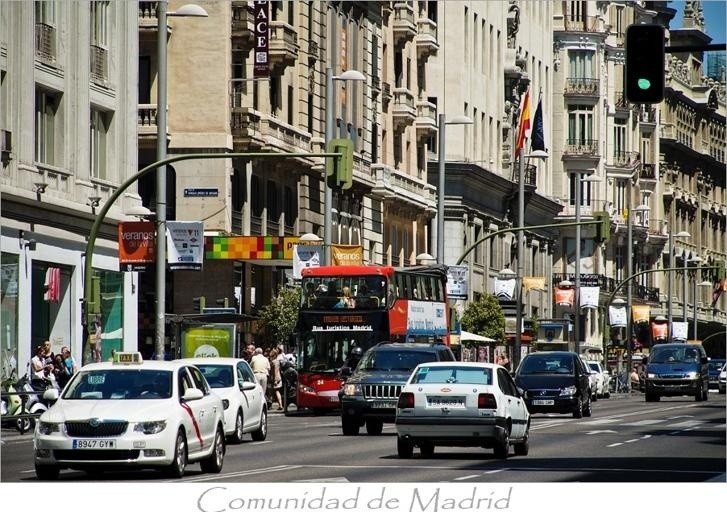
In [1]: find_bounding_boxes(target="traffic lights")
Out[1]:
[624,24,663,102]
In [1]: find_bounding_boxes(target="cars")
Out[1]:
[393,360,530,459]
[512,351,611,418]
[167,357,269,441]
[609,353,727,395]
[33,350,227,479]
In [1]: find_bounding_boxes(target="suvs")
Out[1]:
[337,339,455,435]
[644,342,709,403]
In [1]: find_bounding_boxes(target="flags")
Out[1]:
[531,98,546,156]
[513,86,531,165]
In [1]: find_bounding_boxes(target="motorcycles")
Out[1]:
[1,360,60,432]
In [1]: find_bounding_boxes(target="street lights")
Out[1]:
[156,4,209,359]
[322,66,366,268]
[665,228,692,342]
[574,172,603,354]
[513,147,549,371]
[624,201,651,374]
[682,255,704,321]
[435,113,475,264]
[693,280,715,342]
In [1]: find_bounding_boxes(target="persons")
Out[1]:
[242,345,295,413]
[31,342,73,406]
[629,368,639,382]
[478,348,487,363]
[496,351,511,373]
[302,279,434,376]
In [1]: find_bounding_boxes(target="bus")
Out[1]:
[295,264,451,414]
